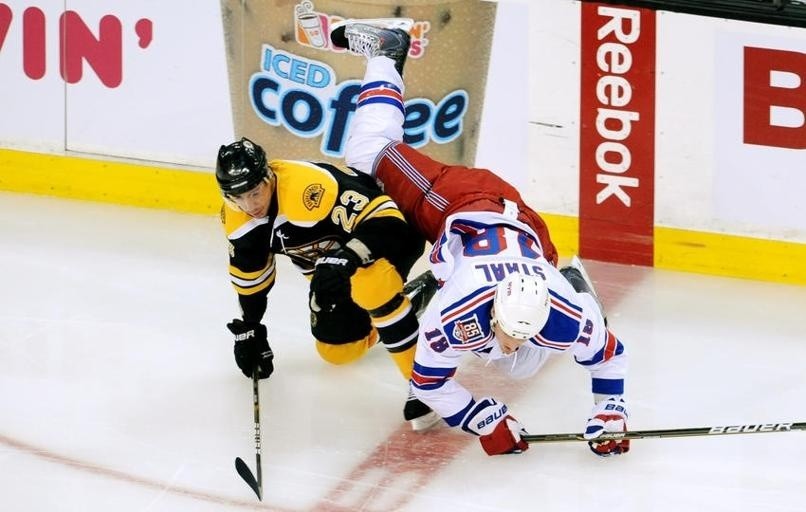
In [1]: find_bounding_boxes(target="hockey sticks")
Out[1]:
[235,364,262,502]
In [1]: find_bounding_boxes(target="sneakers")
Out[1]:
[403,379,434,421]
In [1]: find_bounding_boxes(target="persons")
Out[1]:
[329,24,629,456]
[215,136,430,421]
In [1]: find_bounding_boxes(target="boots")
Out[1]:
[329,21,410,81]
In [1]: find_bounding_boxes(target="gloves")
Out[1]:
[309,245,363,313]
[583,395,630,457]
[459,397,529,455]
[227,318,274,379]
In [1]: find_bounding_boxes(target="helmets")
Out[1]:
[215,137,268,195]
[494,271,551,340]
